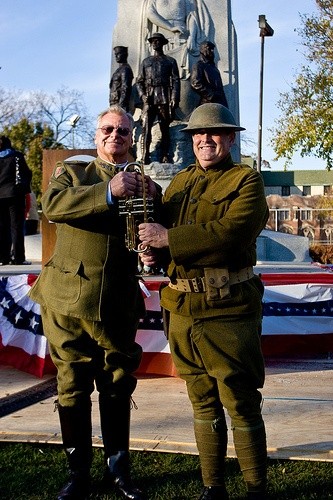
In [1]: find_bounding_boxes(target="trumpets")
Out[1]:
[117,133,166,277]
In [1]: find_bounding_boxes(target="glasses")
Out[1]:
[98,125,132,137]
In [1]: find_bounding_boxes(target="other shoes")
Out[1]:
[23,260,32,265]
[198,486,229,500]
[247,494,264,500]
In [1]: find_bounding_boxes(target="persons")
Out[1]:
[136,33,181,164]
[29,105,162,500]
[136,104,272,500]
[108,46,133,157]
[190,41,229,109]
[145,0,200,81]
[0,135,39,265]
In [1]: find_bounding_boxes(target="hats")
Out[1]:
[178,103,246,132]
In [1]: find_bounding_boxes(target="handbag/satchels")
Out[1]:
[15,156,28,194]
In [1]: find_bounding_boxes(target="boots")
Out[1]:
[54,399,94,500]
[99,394,141,500]
